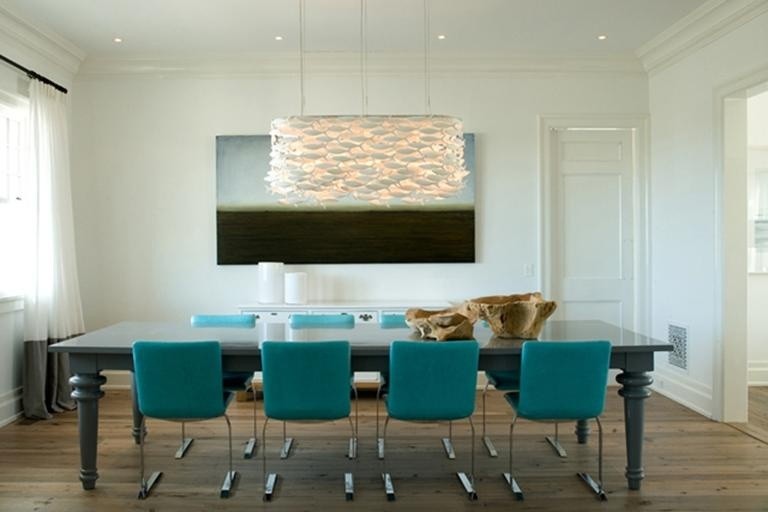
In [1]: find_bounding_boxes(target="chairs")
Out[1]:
[258,340,361,501]
[172,312,260,458]
[499,339,612,503]
[276,313,358,461]
[131,339,238,499]
[378,312,461,461]
[479,319,570,459]
[379,340,482,503]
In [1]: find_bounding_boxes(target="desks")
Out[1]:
[48,320,677,492]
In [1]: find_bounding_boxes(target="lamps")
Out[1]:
[264,0,470,209]
